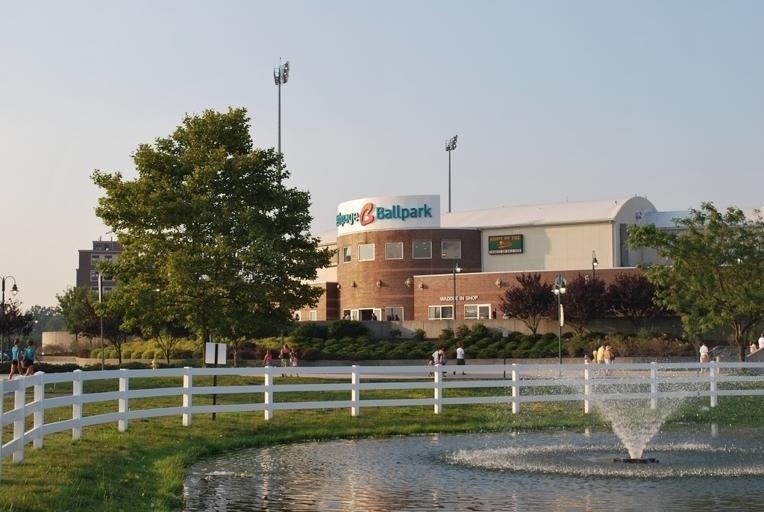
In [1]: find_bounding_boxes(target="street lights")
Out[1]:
[452,259,463,319]
[552,279,567,379]
[270,57,288,187]
[0,274,19,366]
[443,134,458,212]
[592,250,598,280]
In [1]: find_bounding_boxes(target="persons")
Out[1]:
[431,346,439,363]
[277,344,293,377]
[22,340,40,378]
[757,332,764,349]
[426,358,433,376]
[583,344,615,365]
[438,345,447,378]
[492,306,496,319]
[749,340,756,353]
[342,312,399,321]
[699,341,709,374]
[290,353,299,377]
[263,350,272,366]
[452,345,466,375]
[8,339,21,380]
